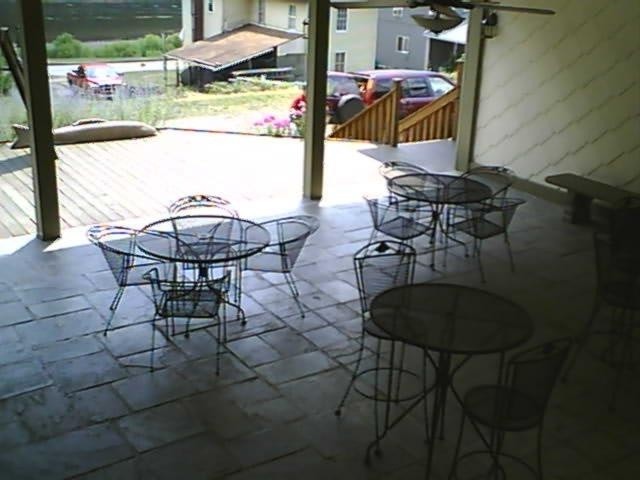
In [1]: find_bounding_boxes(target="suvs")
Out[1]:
[280,65,460,130]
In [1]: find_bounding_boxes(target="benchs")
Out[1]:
[546,174,639,225]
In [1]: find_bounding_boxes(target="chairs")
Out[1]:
[447,336,572,480]
[335,239,433,459]
[142,268,232,377]
[234,214,319,320]
[358,159,529,287]
[168,194,243,313]
[87,224,177,335]
[559,190,640,413]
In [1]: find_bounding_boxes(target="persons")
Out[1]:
[288,85,307,132]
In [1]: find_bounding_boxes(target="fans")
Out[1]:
[328,0,558,32]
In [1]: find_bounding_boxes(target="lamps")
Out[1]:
[480,12,497,41]
[413,9,465,36]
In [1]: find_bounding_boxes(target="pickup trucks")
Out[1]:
[66,58,130,101]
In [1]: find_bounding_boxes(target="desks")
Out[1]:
[364,282,534,479]
[135,214,271,339]
